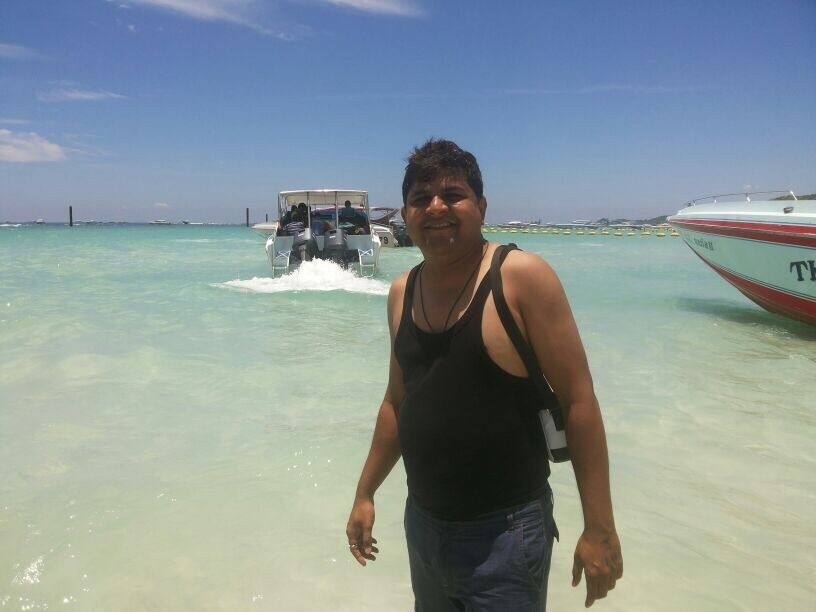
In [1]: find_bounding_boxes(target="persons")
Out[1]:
[283,202,307,234]
[339,201,356,223]
[346,137,623,612]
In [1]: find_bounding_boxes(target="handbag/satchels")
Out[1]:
[490,246,572,464]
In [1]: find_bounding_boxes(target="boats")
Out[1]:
[149,218,171,225]
[35,217,45,224]
[250,189,412,279]
[665,189,816,326]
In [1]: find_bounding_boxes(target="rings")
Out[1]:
[349,544,360,548]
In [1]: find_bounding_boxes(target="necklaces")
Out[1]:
[417,239,489,360]
